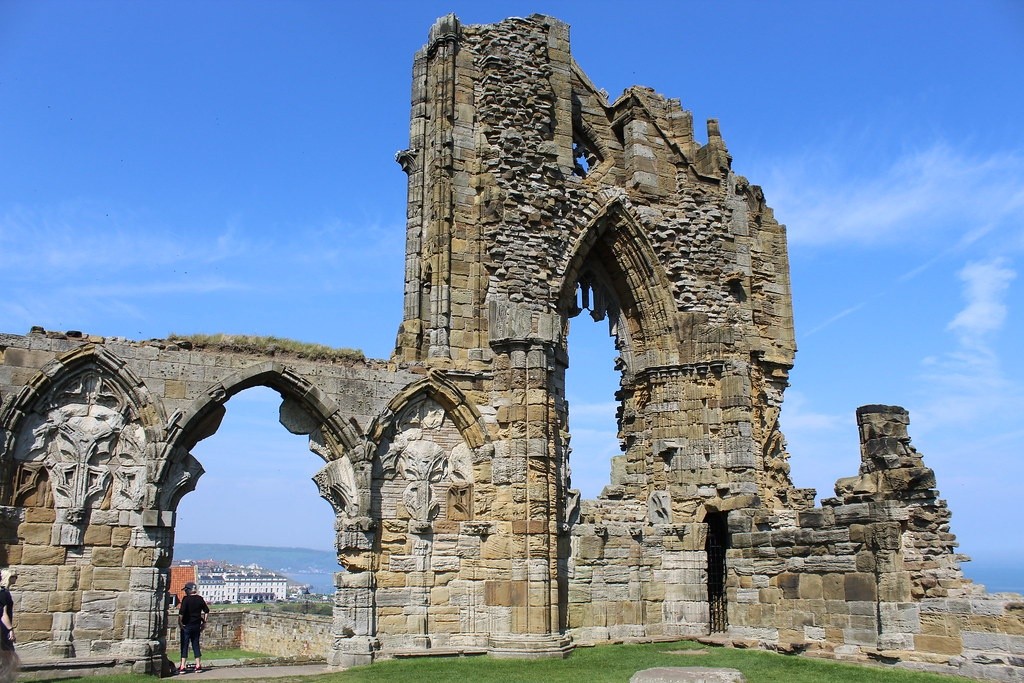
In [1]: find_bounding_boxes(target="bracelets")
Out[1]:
[8,627,13,630]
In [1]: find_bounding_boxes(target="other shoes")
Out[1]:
[176,666,186,674]
[192,666,202,673]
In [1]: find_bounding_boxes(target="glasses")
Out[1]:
[11,575,18,579]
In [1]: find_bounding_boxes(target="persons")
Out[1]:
[0,568,23,683]
[174,582,210,675]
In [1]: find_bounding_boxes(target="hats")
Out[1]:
[180,582,197,592]
